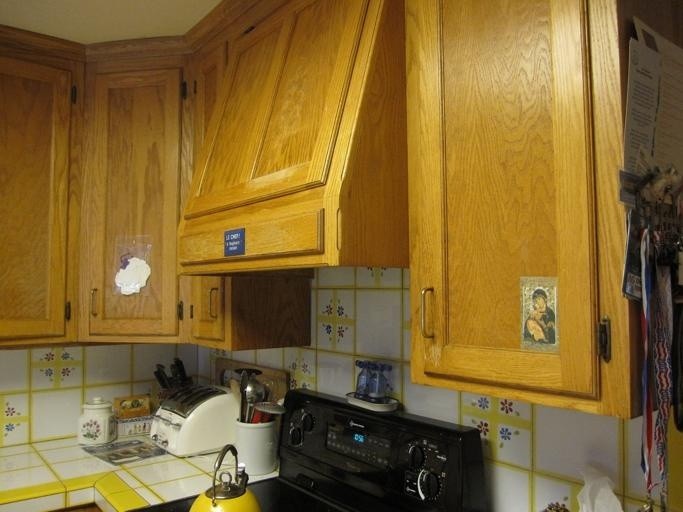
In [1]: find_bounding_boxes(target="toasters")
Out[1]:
[149,383,239,459]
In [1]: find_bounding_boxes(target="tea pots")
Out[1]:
[188,444,261,512]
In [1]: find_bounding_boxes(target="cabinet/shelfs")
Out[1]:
[174,0,409,275]
[0,24,85,351]
[76,34,184,344]
[405,0,630,420]
[179,14,311,351]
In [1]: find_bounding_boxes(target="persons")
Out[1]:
[524,289,555,345]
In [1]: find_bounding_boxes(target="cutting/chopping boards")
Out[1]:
[215,358,288,408]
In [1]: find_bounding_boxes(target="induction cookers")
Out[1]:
[110,476,433,512]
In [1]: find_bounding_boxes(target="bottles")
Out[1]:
[354,359,392,403]
[75,395,116,446]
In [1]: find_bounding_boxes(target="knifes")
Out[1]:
[152,357,194,391]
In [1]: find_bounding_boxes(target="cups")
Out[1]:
[235,417,279,477]
